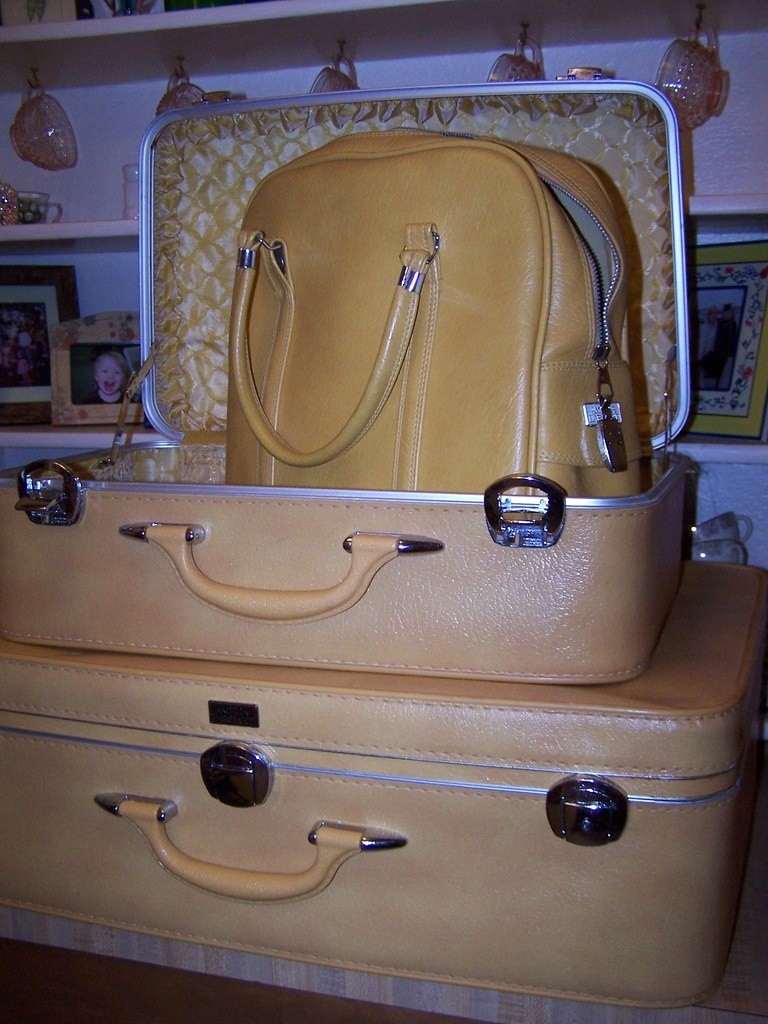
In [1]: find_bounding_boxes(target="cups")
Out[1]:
[487,35,548,86]
[10,82,78,171]
[153,71,206,119]
[689,510,754,565]
[652,21,730,134]
[15,189,63,224]
[308,52,359,95]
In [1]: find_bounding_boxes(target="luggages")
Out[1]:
[0,562,768,1003]
[0,79,689,684]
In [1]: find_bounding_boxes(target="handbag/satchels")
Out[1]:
[224,131,649,496]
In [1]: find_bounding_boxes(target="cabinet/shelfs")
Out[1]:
[0,2,768,243]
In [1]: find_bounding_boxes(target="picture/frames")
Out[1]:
[1,264,81,427]
[682,239,767,447]
[48,311,146,427]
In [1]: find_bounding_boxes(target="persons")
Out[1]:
[80,350,135,407]
[687,301,743,393]
